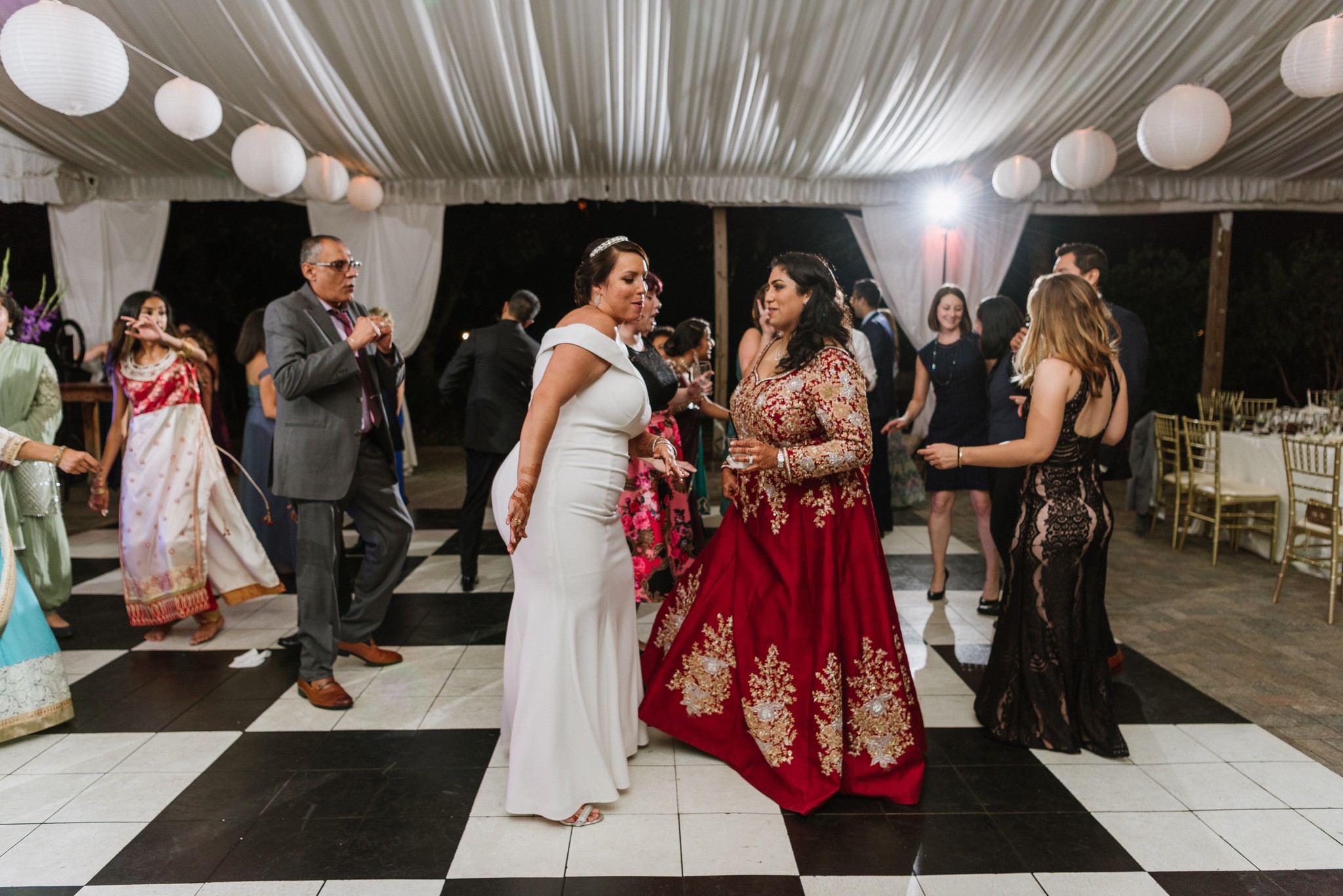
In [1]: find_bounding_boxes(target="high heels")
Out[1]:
[977,577,1004,612]
[927,567,949,600]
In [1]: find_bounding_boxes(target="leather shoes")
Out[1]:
[296,673,354,710]
[338,639,402,666]
[461,575,480,592]
[1106,647,1125,677]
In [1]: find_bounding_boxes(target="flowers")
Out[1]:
[2,248,67,344]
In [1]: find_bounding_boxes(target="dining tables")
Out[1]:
[1257,407,1338,427]
[1188,429,1343,584]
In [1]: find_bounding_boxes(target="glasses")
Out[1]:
[309,261,362,272]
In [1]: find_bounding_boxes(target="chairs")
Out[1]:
[1198,394,1238,433]
[1151,414,1228,551]
[1209,389,1245,434]
[1177,416,1281,567]
[1231,396,1277,431]
[1323,401,1343,435]
[1273,434,1342,627]
[1308,390,1340,407]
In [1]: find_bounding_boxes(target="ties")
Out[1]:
[332,311,382,427]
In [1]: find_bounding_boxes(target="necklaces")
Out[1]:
[774,335,795,361]
[932,333,965,387]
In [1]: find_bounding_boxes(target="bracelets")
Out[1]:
[173,334,194,359]
[53,441,68,467]
[347,336,358,353]
[88,475,110,498]
[651,436,677,458]
[957,445,961,468]
[959,446,964,470]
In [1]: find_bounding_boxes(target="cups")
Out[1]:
[728,434,757,468]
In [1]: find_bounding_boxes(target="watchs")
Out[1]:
[776,446,785,471]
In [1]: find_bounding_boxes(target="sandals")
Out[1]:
[557,802,604,827]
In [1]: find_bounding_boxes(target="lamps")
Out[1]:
[1280,18,1343,98]
[155,76,224,141]
[229,124,308,198]
[1051,129,1118,190]
[992,155,1042,200]
[347,174,384,212]
[301,154,349,203]
[0,0,131,116]
[1136,84,1232,172]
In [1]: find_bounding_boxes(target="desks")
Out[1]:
[59,382,118,488]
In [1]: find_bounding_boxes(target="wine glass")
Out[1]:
[694,361,714,396]
[1233,401,1342,442]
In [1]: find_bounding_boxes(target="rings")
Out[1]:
[512,518,522,526]
[930,460,934,466]
[748,456,754,465]
[378,321,385,328]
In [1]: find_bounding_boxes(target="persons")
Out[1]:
[0,423,104,743]
[232,306,308,579]
[486,232,685,827]
[717,281,776,519]
[915,271,1133,759]
[837,326,876,392]
[638,251,930,814]
[879,283,1004,615]
[439,288,554,592]
[1010,240,1143,675]
[613,272,714,614]
[0,293,78,640]
[662,316,737,553]
[973,294,1031,630]
[845,277,894,539]
[83,290,228,645]
[262,232,417,709]
